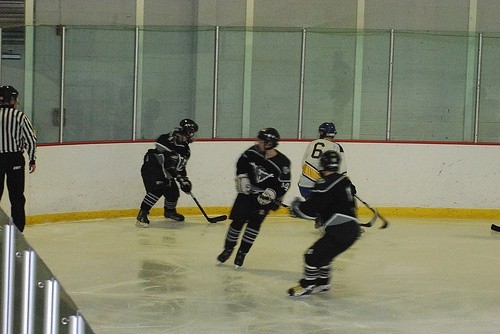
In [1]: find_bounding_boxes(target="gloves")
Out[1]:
[257,188,276,205]
[235,173,252,195]
[176,176,193,193]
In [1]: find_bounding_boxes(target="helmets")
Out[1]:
[180,118,199,132]
[318,122,337,137]
[0,85,19,98]
[320,150,342,171]
[257,127,280,151]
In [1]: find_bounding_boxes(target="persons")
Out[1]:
[288,150,361,297]
[298,122,346,227]
[135,119,198,224]
[217,128,290,265]
[0,85,36,232]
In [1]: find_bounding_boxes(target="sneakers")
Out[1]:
[163,207,185,223]
[217,248,234,265]
[135,209,150,228]
[234,249,247,270]
[286,277,332,297]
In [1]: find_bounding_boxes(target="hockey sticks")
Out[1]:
[354,194,461,230]
[490,223,500,232]
[176,177,228,224]
[247,191,377,228]
